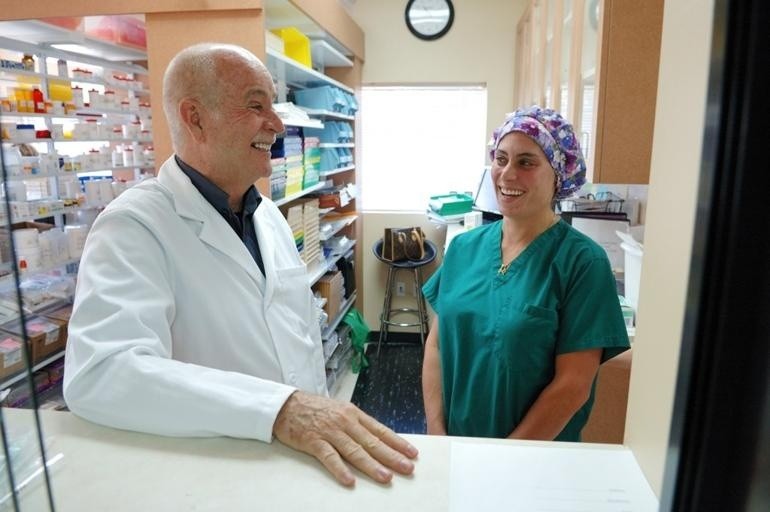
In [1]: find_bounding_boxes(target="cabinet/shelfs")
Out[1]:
[3,17,153,417]
[145,13,371,404]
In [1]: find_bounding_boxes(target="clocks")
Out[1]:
[404,0,455,39]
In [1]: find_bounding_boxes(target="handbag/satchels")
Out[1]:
[382,226,428,264]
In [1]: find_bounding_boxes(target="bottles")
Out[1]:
[32,85,44,112]
[22,53,34,71]
[54,58,154,206]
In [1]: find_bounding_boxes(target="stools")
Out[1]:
[371,236,437,361]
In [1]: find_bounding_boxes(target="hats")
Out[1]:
[486,105,586,202]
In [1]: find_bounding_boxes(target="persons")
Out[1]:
[421,105,631,441]
[63,43,419,486]
